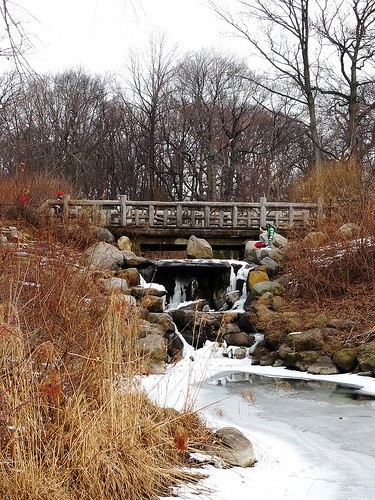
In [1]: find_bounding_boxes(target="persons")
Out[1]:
[254,223,278,250]
[53,192,64,218]
[19,187,31,210]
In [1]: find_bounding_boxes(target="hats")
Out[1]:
[57,191,64,197]
